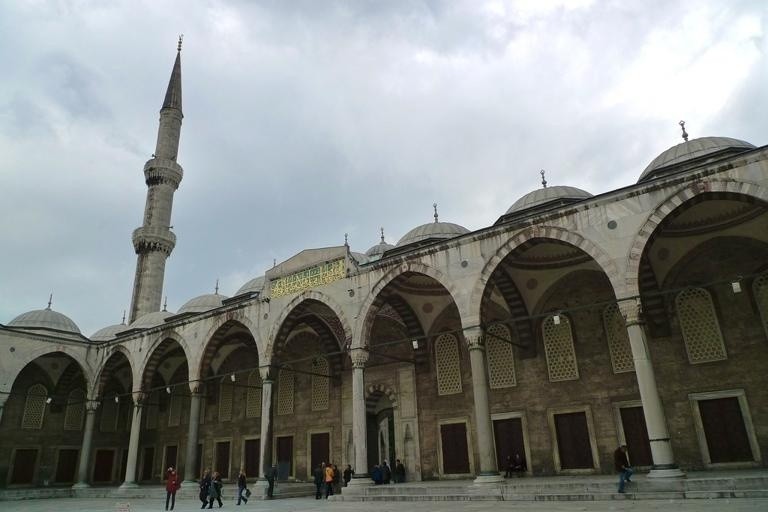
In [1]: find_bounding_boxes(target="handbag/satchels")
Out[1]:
[241,489,251,498]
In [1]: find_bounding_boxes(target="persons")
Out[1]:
[166,466,177,510]
[314,461,354,499]
[199,470,224,509]
[236,472,247,505]
[504,454,524,478]
[372,459,405,485]
[266,464,279,497]
[615,445,633,493]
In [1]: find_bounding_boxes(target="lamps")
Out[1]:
[263,371,275,384]
